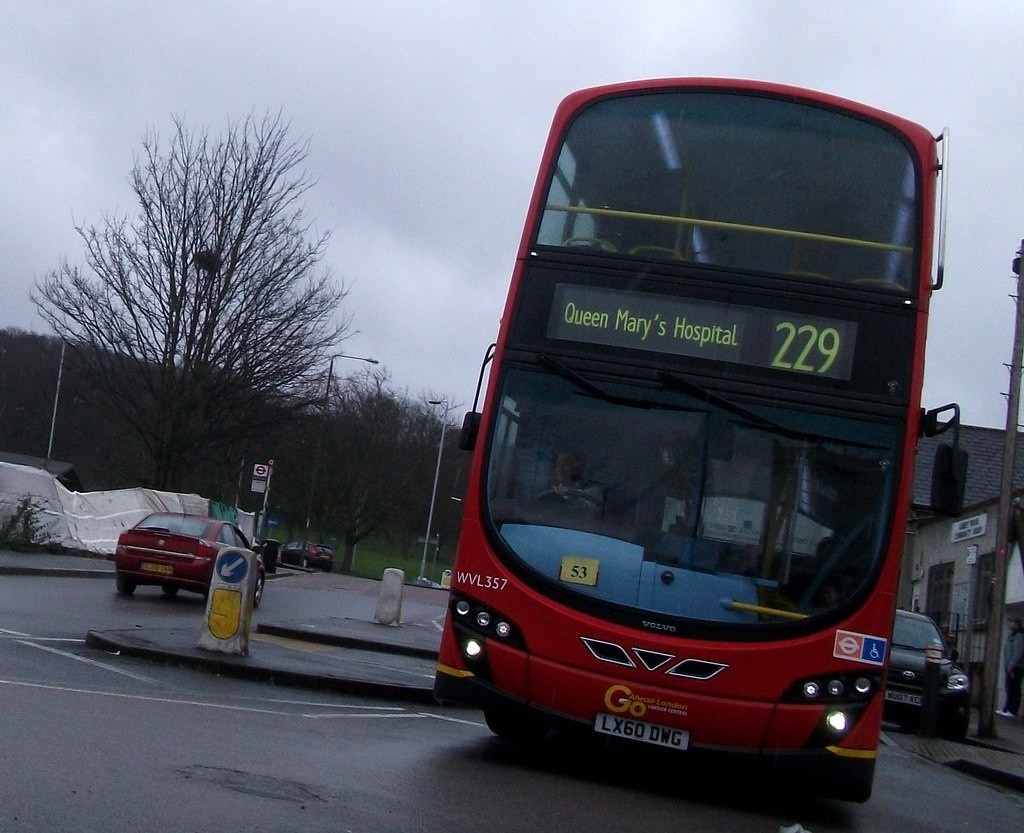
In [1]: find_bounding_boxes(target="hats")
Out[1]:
[1008,616,1021,623]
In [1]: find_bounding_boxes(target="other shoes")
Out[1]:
[994,708,1016,719]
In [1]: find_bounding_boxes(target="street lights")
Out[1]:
[305,355,380,542]
[417,401,449,582]
[46,337,66,461]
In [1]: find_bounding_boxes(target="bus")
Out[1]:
[430,75,970,808]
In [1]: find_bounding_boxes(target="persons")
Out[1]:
[996,617,1024,718]
[547,450,604,513]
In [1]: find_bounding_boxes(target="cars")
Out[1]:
[281,541,334,572]
[881,608,970,741]
[116,510,265,610]
